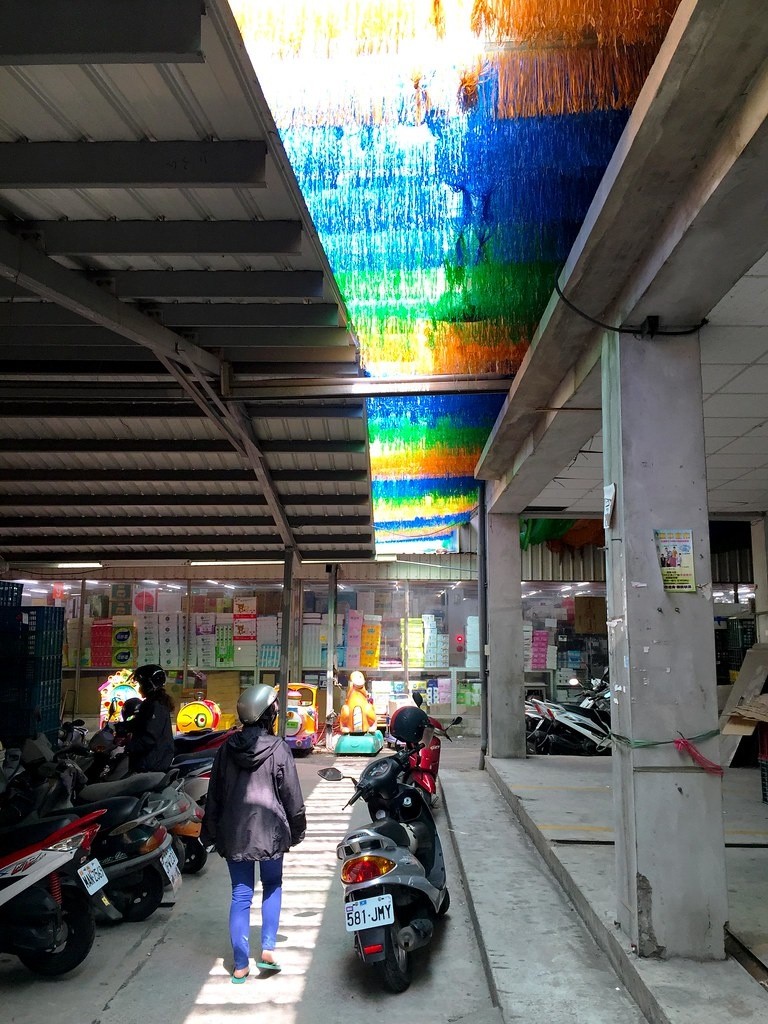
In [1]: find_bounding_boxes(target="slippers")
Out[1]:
[257,959,281,971]
[232,965,251,984]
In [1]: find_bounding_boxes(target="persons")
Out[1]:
[116,664,174,776]
[660,547,682,567]
[202,684,307,984]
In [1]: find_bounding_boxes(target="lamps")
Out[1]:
[98,560,191,567]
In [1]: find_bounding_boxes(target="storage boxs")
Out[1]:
[207,671,274,731]
[715,617,758,685]
[757,721,768,805]
[557,672,577,685]
[523,605,588,669]
[0,581,65,752]
[163,671,207,722]
[305,673,338,689]
[31,583,441,619]
[401,614,449,667]
[463,616,480,668]
[62,597,257,666]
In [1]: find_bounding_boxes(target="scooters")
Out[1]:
[400,714,463,810]
[0,699,244,979]
[522,665,612,756]
[316,736,452,995]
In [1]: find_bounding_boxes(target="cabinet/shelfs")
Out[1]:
[61,667,589,718]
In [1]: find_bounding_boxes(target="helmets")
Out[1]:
[237,684,278,724]
[134,665,166,694]
[399,822,419,854]
[121,697,142,721]
[390,706,428,743]
